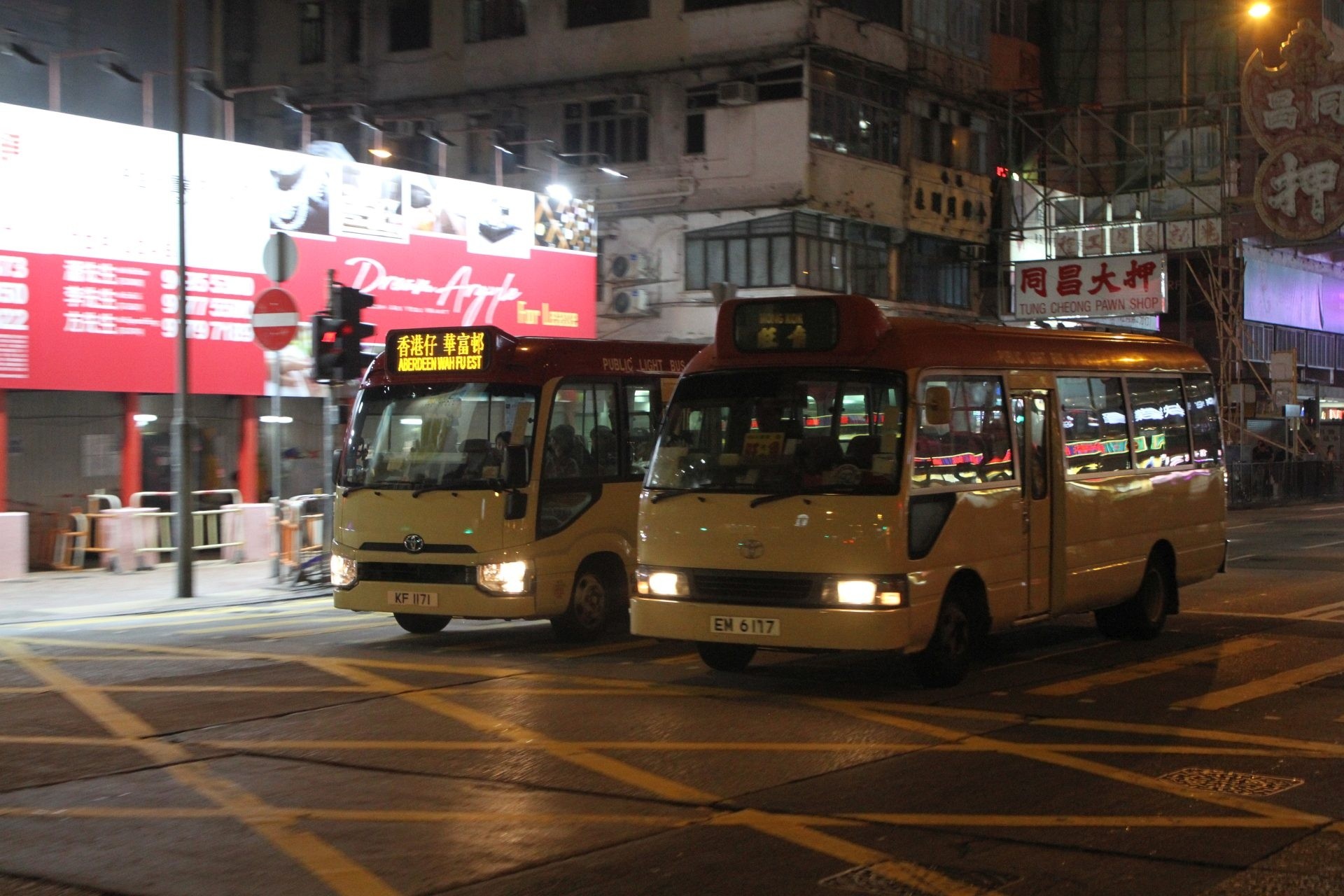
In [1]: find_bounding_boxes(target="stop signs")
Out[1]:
[251,287,299,353]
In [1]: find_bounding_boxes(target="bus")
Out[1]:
[628,294,1230,693]
[330,326,708,648]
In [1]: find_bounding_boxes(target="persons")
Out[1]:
[487,422,655,484]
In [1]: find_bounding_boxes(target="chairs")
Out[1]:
[793,430,1210,471]
[452,433,659,484]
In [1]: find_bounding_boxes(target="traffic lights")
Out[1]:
[333,287,376,370]
[311,314,348,378]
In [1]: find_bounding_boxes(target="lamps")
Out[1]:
[0,29,628,200]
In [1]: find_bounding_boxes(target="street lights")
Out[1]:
[1180,1,1271,126]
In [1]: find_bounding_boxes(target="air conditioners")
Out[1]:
[603,250,651,317]
[616,93,646,116]
[716,82,757,105]
